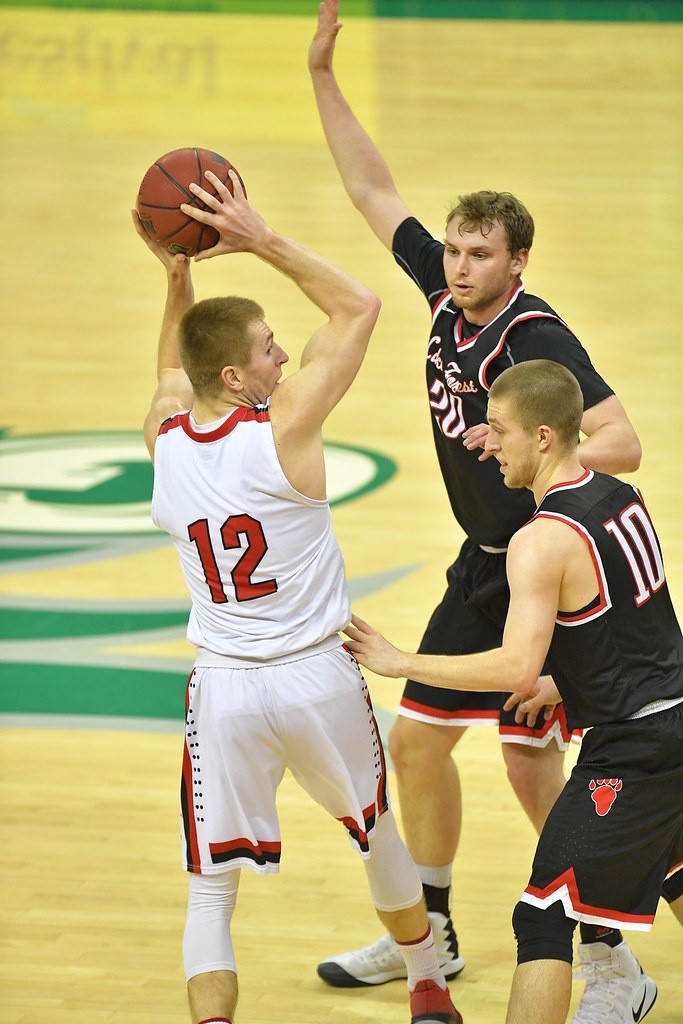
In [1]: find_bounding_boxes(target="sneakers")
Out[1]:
[410,979,463,1024]
[570,939,659,1024]
[317,911,465,988]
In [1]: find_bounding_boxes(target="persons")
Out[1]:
[308,0,658,1022]
[341,359,683,1024]
[128,167,467,1024]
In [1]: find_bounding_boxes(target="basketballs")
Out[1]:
[137,147,244,260]
